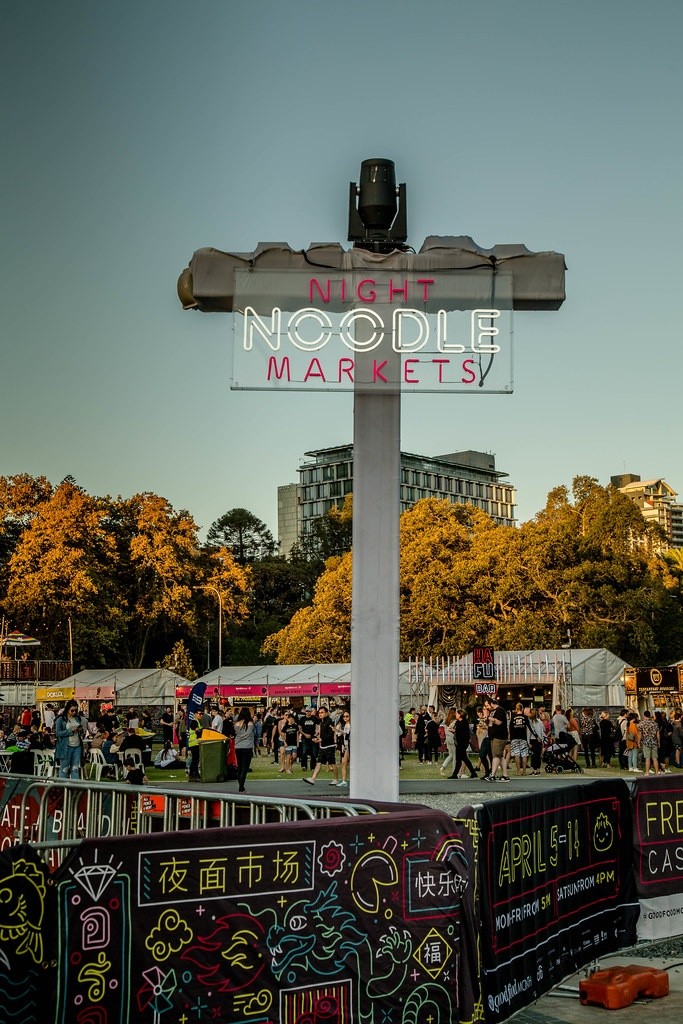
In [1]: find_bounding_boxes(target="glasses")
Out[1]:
[198,713,204,715]
[343,716,349,718]
[306,711,310,712]
[71,709,78,713]
[286,714,287,716]
[319,712,324,714]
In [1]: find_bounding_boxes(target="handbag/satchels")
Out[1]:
[628,732,635,742]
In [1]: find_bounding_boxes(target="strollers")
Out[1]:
[540,736,585,774]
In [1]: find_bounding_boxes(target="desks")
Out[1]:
[117,752,124,763]
[0,750,14,773]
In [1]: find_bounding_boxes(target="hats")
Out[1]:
[306,707,312,711]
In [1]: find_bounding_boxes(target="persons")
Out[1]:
[400,700,683,782]
[23,649,30,662]
[0,697,352,793]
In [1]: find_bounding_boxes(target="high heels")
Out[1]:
[470,773,478,778]
[448,776,458,779]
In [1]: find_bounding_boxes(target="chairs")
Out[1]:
[30,749,60,777]
[88,748,118,781]
[123,749,144,778]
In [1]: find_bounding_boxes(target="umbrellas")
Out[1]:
[0,626,41,660]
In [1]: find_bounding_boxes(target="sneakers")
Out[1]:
[538,772,541,776]
[485,774,496,781]
[495,776,511,782]
[529,771,539,776]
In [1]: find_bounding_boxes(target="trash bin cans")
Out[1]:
[197,728,229,783]
[136,728,156,766]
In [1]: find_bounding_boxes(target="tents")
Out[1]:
[623,660,683,721]
[36,667,191,741]
[430,648,634,715]
[174,661,436,713]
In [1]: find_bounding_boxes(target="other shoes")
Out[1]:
[269,762,279,765]
[337,782,347,787]
[461,774,469,778]
[665,769,672,773]
[629,767,634,771]
[329,781,340,785]
[634,768,643,772]
[649,769,655,773]
[474,766,480,771]
[303,778,315,785]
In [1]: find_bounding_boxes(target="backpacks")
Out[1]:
[609,719,626,742]
